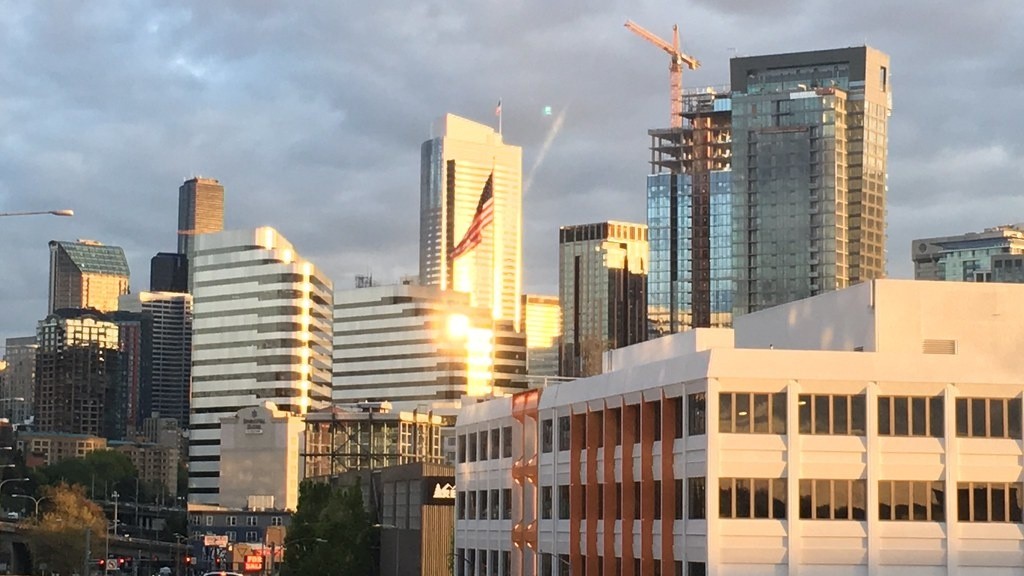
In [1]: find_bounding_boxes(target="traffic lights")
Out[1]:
[216,558,221,564]
[185,556,191,563]
[99,559,106,569]
[120,558,125,564]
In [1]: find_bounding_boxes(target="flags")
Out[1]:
[495,99,501,115]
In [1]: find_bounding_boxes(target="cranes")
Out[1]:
[622,19,702,127]
